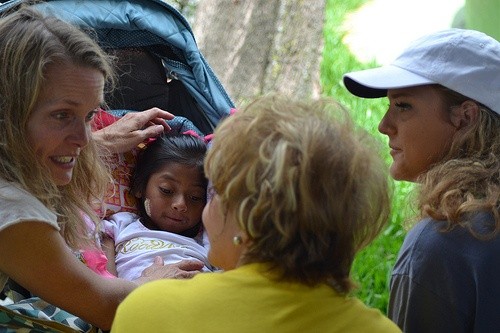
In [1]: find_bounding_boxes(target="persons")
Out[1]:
[98,130,208,280]
[0,5,204,330]
[343,29,500,333]
[110,93,402,333]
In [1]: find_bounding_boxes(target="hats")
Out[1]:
[342,25,499,117]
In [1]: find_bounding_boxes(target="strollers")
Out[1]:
[0,0,238,333]
